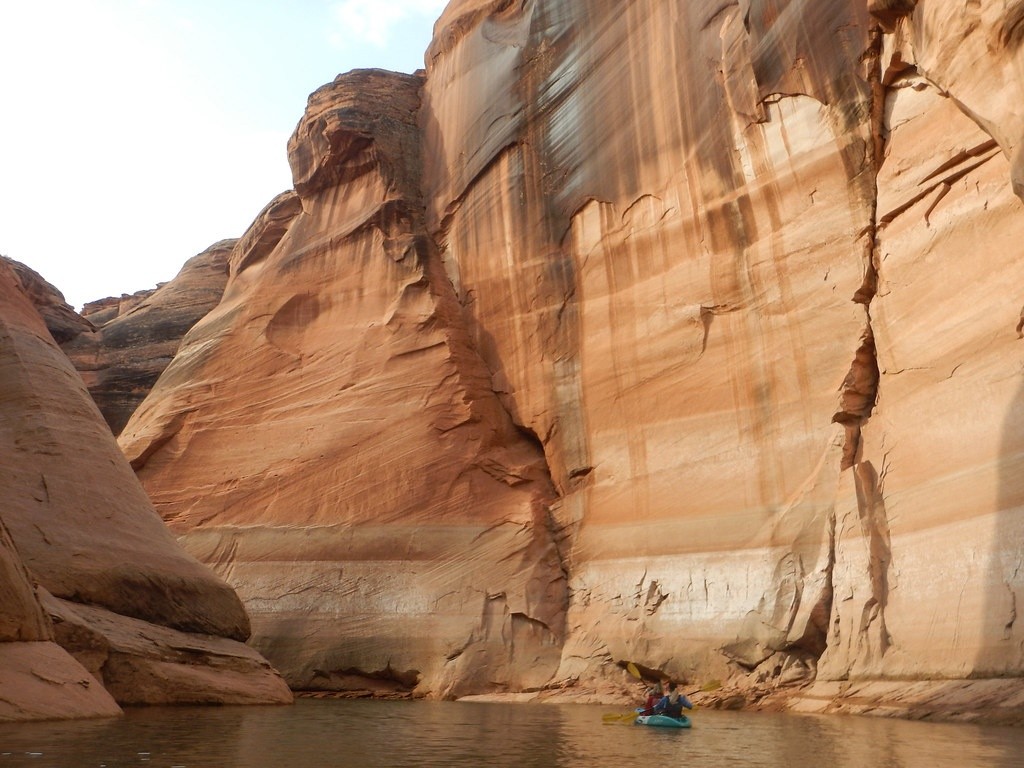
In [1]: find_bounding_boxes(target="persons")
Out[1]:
[643,681,664,715]
[653,683,692,719]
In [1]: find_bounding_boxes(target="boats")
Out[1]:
[633,706,692,729]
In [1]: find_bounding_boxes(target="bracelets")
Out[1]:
[646,689,648,691]
